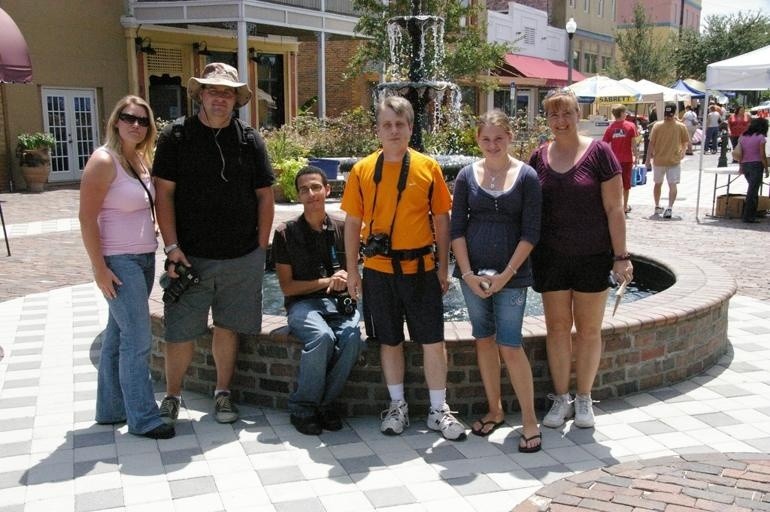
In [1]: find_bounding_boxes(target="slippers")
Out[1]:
[518,429,542,452]
[473,420,504,436]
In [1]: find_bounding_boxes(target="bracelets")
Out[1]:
[505,263,518,275]
[609,253,635,261]
[163,244,183,255]
[460,269,474,280]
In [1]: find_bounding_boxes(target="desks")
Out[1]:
[704,163,769,219]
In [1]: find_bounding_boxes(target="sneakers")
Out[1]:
[380,401,409,435]
[160,396,180,419]
[543,393,574,427]
[141,424,175,439]
[215,392,238,422]
[655,206,672,218]
[291,408,341,435]
[573,392,600,427]
[427,403,466,440]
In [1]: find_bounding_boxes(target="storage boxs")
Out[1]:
[715,193,770,219]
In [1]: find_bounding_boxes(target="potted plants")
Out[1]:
[16,129,56,192]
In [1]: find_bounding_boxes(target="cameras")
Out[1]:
[364,233,388,257]
[337,293,356,315]
[474,266,499,290]
[163,266,200,303]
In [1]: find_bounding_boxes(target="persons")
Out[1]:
[348,96,466,442]
[77,92,181,440]
[526,87,634,429]
[153,62,275,427]
[450,110,549,453]
[273,165,360,437]
[603,100,768,224]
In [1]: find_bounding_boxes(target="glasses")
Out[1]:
[119,113,150,127]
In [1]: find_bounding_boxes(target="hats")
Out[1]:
[187,63,252,109]
[665,103,676,117]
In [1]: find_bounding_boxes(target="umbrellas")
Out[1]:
[0,6,33,259]
[558,72,768,117]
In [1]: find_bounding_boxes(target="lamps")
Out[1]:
[135,35,156,55]
[249,47,263,63]
[193,40,212,57]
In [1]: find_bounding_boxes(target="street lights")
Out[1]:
[564,16,578,87]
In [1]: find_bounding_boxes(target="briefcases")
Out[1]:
[631,164,646,186]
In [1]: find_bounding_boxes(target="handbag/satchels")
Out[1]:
[732,143,742,162]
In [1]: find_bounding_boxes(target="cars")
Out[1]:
[751,100,770,116]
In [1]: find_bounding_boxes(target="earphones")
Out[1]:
[201,92,203,98]
